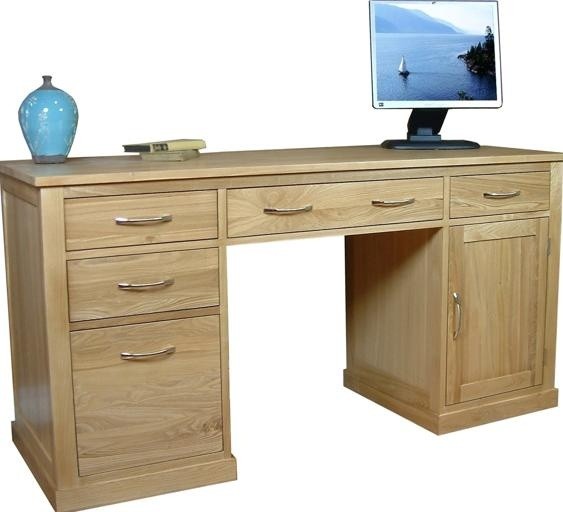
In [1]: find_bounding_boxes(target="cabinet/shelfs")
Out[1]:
[0,140,563,512]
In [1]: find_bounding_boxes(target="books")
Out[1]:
[122,139,206,152]
[141,149,200,162]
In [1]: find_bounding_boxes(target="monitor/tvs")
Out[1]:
[369,0,503,150]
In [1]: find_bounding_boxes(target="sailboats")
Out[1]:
[397,56,409,78]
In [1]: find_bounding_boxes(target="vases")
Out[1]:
[11,72,83,166]
